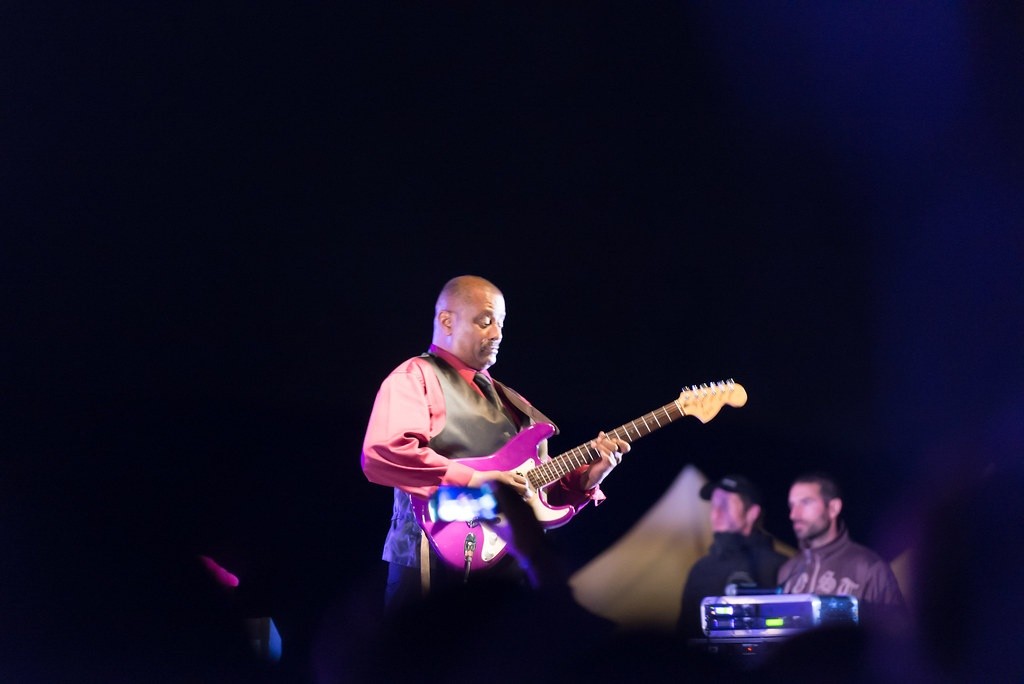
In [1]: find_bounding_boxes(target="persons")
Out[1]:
[680,470,930,684]
[359,275,631,604]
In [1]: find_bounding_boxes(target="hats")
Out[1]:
[699,473,752,500]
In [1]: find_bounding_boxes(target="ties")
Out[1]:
[473,373,500,410]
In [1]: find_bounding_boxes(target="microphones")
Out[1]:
[725,583,784,596]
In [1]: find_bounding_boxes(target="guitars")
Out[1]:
[407,377,749,572]
